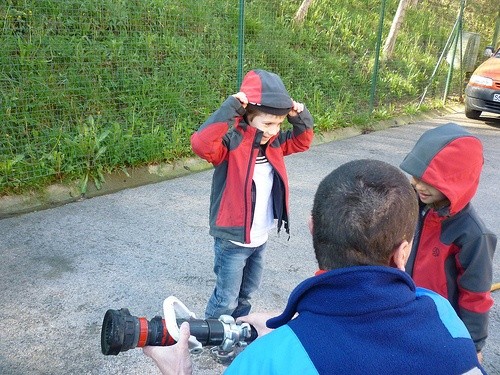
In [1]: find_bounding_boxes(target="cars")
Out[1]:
[464,46,500,121]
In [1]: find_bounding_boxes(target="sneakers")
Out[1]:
[209,346,235,364]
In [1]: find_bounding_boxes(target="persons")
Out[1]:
[190,69,314,321]
[400,122,498,365]
[144,159,487,375]
